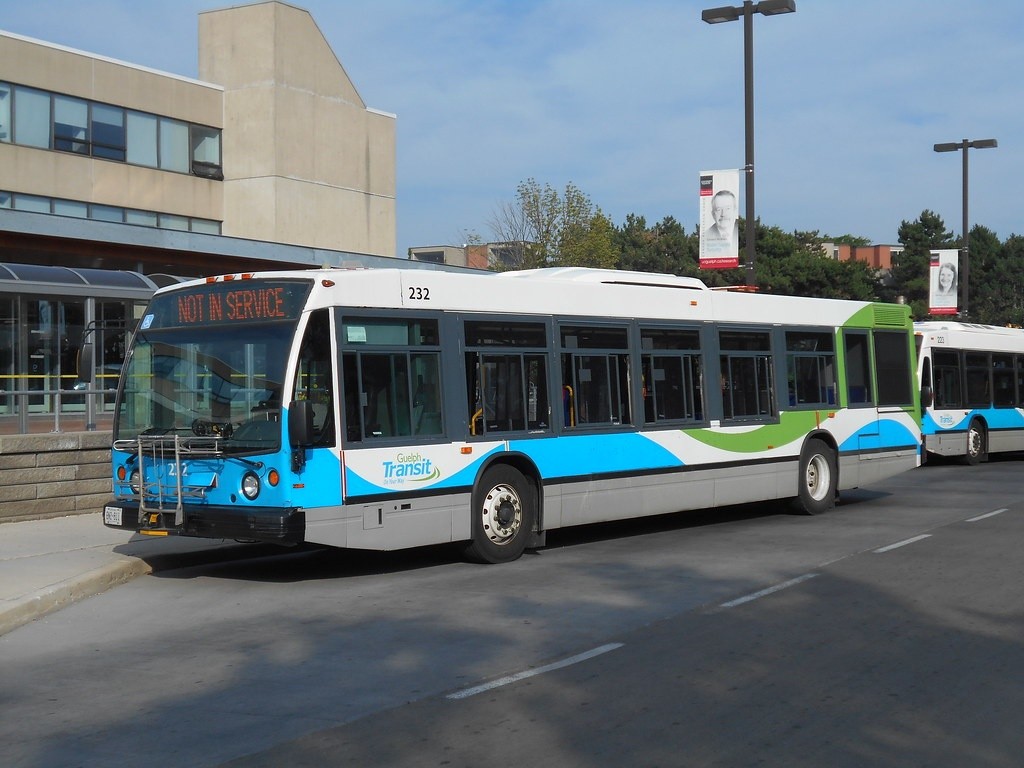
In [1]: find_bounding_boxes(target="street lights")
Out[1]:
[700,0,797,294]
[932,138,998,324]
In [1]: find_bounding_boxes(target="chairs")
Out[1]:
[314,379,441,445]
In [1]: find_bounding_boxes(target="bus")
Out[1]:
[811,321,1024,466]
[75,266,923,565]
